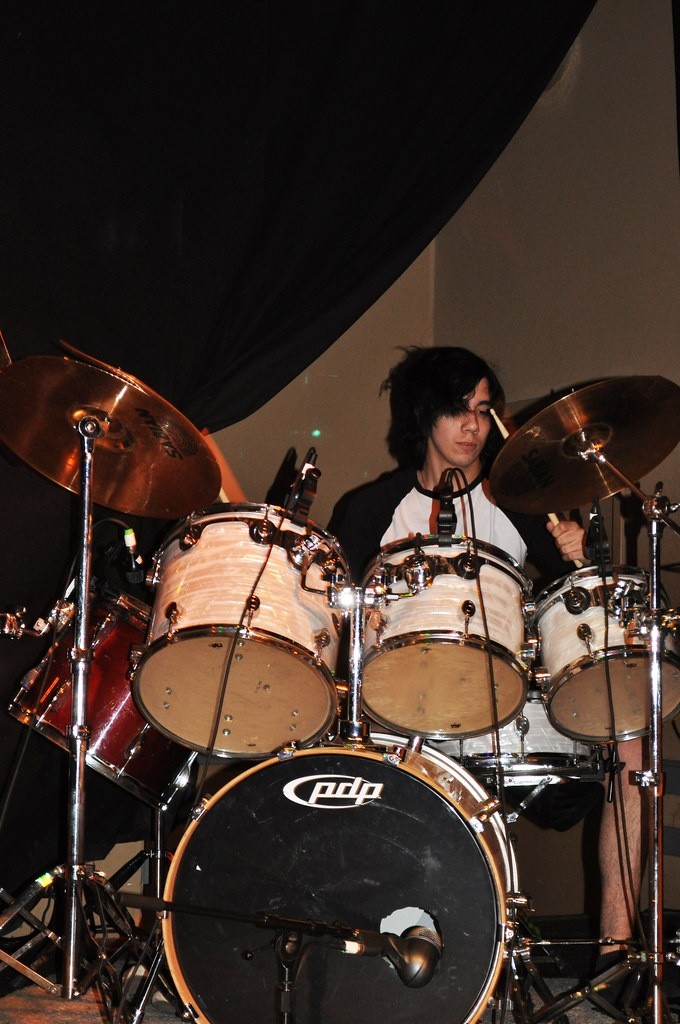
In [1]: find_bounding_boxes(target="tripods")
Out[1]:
[523,442,680,1024]
[0,416,192,1023]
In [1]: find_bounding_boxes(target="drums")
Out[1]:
[125,502,352,763]
[10,581,203,811]
[158,732,523,1024]
[537,565,680,743]
[358,534,533,743]
[426,680,609,787]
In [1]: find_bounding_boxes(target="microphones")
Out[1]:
[351,925,443,988]
[122,529,146,583]
[583,498,600,561]
[437,482,456,534]
[283,453,318,510]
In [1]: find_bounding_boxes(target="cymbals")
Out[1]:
[487,375,680,513]
[1,357,223,520]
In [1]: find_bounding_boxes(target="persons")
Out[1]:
[151,344,652,1013]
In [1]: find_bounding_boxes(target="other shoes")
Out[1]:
[590,970,680,1010]
